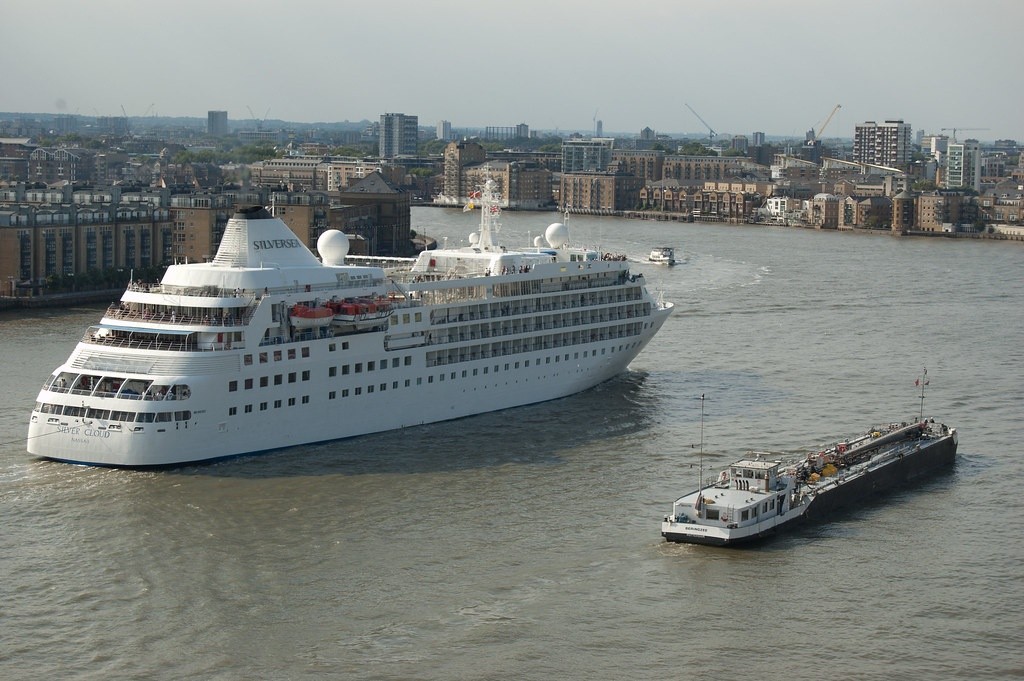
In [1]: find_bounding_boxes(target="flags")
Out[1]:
[462,202,471,213]
[470,190,481,199]
[469,203,474,210]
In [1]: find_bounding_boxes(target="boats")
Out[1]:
[649,246,675,267]
[289,305,334,328]
[25,161,676,469]
[326,295,396,327]
[658,365,960,552]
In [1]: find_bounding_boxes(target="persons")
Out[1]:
[914,416,919,423]
[41,231,676,465]
[676,421,914,532]
[929,416,934,424]
[923,418,929,423]
[941,423,948,431]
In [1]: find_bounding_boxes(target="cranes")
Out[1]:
[683,103,718,147]
[808,103,843,146]
[941,127,991,138]
